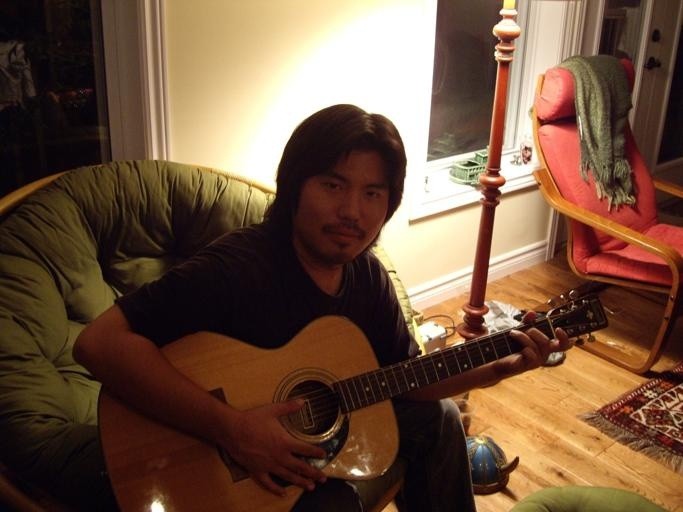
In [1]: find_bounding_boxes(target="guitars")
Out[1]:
[97,282,609,512]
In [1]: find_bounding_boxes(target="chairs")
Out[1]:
[528,56,681,374]
[0,159,418,511]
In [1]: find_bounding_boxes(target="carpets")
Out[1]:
[584,363,682,475]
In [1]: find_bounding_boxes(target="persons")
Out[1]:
[72,104,572,512]
